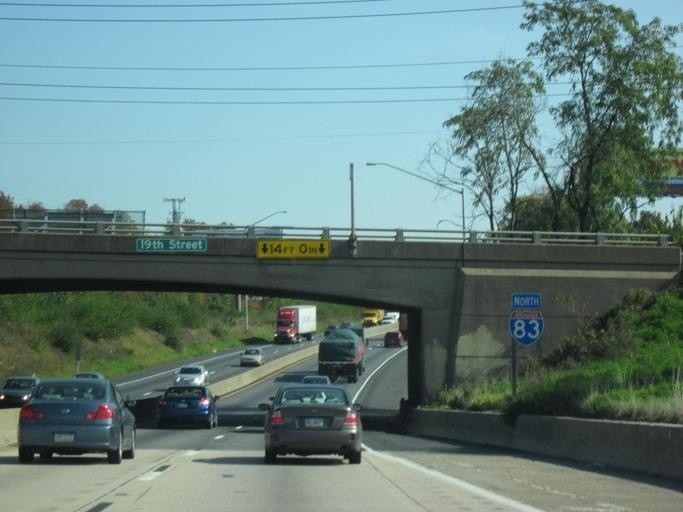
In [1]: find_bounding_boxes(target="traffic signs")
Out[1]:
[511,294,542,310]
[254,236,329,260]
[509,309,545,346]
[135,236,208,252]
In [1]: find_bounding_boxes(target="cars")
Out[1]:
[323,321,354,336]
[0,377,38,408]
[380,312,400,325]
[259,375,362,466]
[174,365,210,387]
[158,386,220,429]
[17,379,137,465]
[76,372,104,380]
[385,332,404,348]
[239,348,264,367]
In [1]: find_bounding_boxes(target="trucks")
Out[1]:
[273,305,317,342]
[361,308,385,327]
[317,326,367,383]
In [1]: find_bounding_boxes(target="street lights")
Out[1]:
[366,162,465,243]
[253,210,287,226]
